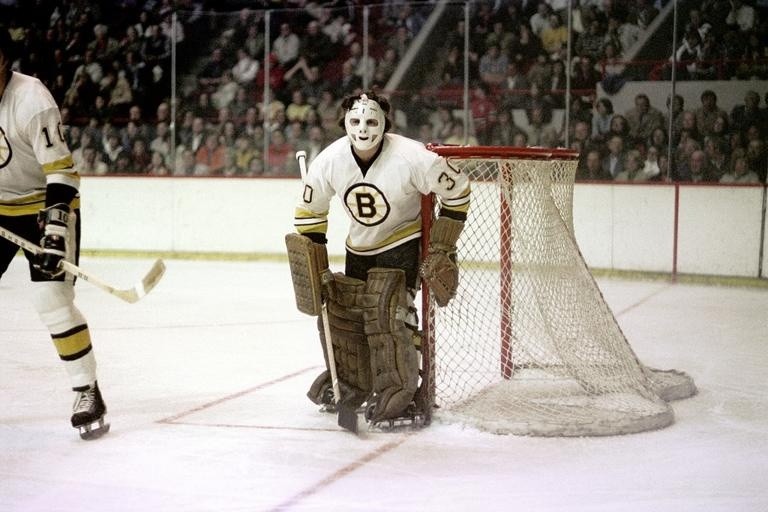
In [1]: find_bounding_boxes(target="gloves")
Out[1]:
[319,269,336,302]
[28,203,72,277]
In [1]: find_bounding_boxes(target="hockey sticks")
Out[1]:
[295,149,343,415]
[0,224,165,304]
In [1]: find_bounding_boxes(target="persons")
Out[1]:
[292,91,471,432]
[468,2,674,182]
[1,1,265,179]
[269,2,464,179]
[673,0,768,185]
[0,25,106,428]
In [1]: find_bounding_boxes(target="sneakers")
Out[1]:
[393,397,418,420]
[321,385,336,405]
[70,380,106,427]
